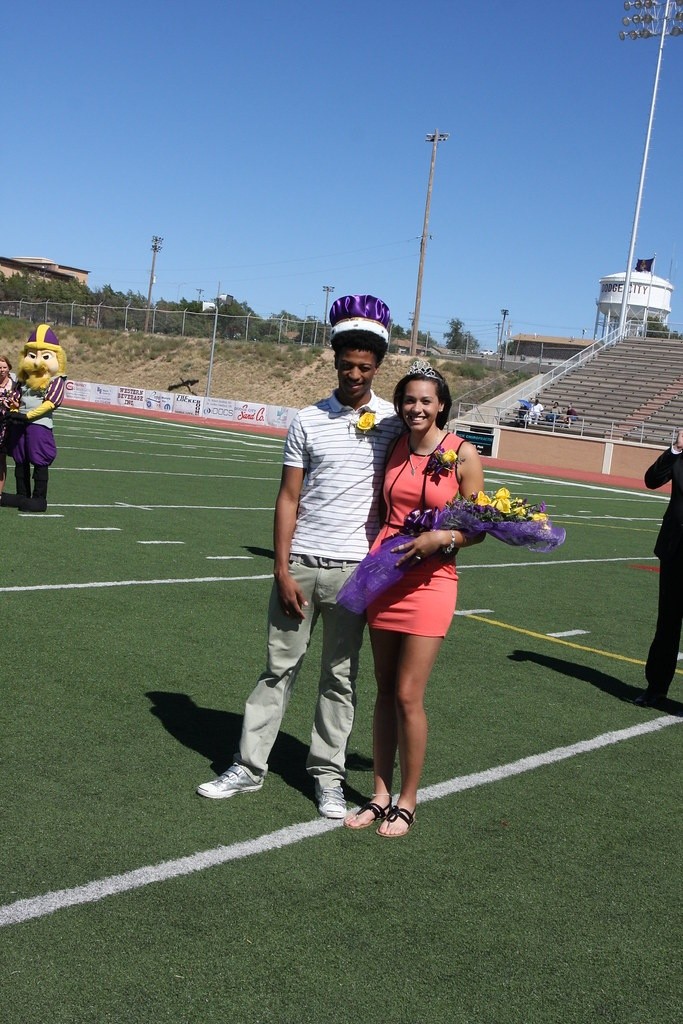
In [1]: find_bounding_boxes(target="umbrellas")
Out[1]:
[518,400,532,409]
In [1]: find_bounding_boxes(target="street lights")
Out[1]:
[619,0,683,328]
[143,234,163,332]
[322,285,335,347]
[410,128,450,359]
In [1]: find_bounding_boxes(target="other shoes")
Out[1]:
[635,687,667,706]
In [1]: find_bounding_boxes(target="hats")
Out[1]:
[329,294,391,348]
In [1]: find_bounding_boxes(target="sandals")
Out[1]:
[344,796,392,830]
[375,804,416,837]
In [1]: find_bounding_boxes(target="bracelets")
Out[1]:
[443,530,455,554]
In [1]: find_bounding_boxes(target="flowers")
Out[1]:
[356,412,384,435]
[424,444,465,476]
[335,487,568,614]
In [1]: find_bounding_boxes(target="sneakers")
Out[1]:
[196,764,264,799]
[315,783,347,818]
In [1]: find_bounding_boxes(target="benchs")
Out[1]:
[499,337,683,446]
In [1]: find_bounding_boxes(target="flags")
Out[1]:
[635,258,654,272]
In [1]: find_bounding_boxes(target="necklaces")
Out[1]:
[409,428,441,474]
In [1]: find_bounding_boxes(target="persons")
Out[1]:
[344,362,485,838]
[198,295,407,818]
[634,426,683,705]
[0,355,18,503]
[518,399,578,427]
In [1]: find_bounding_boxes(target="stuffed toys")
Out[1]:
[3,323,67,512]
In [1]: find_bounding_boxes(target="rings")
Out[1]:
[416,556,423,560]
